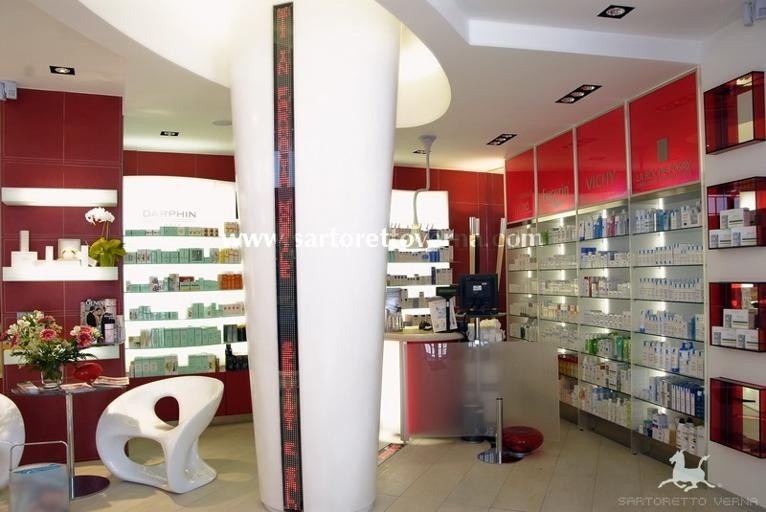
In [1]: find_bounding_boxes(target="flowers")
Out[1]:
[1,310,103,379]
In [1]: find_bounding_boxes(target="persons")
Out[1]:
[87,305,113,342]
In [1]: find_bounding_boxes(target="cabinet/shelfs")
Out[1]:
[574,101,631,450]
[627,65,708,479]
[504,147,537,342]
[702,71,766,463]
[535,126,579,427]
[379,327,464,446]
[388,237,462,327]
[124,233,252,421]
[1,187,119,363]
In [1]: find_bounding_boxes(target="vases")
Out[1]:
[100,253,115,265]
[40,366,63,388]
[84,208,127,258]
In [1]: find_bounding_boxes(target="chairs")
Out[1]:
[94,375,225,494]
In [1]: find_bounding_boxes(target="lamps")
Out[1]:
[406,135,437,249]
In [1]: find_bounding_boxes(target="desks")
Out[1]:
[11,374,118,500]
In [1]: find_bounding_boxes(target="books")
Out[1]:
[92,375,129,388]
[58,382,95,393]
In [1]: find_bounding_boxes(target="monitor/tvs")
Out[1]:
[458,273,498,307]
